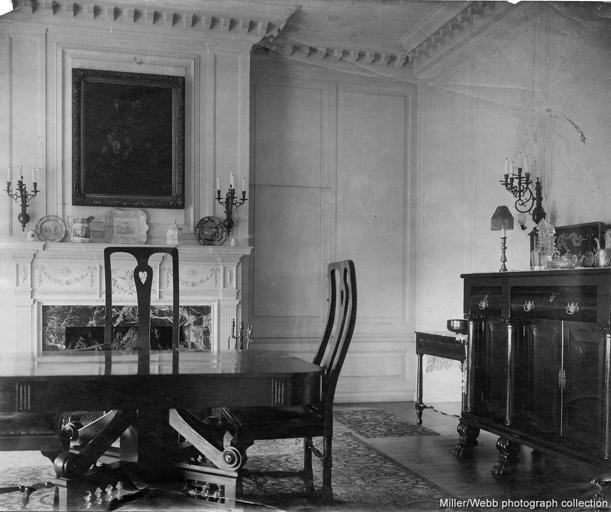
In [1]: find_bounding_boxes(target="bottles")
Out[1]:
[166,218,183,247]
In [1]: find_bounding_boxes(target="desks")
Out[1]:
[0,351,322,512]
[414,329,468,425]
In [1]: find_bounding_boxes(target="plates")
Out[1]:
[34,214,66,242]
[101,207,150,245]
[195,215,228,246]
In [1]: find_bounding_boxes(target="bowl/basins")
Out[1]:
[446,319,468,332]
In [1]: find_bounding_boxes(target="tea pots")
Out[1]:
[555,235,609,268]
[65,214,96,243]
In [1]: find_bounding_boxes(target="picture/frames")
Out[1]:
[71,67,186,210]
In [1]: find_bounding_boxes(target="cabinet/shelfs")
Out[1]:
[451,269,610,498]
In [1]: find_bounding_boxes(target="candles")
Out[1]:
[505,151,529,174]
[216,170,247,192]
[8,162,37,183]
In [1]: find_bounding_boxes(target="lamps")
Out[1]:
[491,205,514,272]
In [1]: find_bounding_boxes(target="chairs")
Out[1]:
[104,246,179,350]
[217,260,357,502]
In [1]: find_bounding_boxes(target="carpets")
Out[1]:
[0,431,481,512]
[333,409,439,438]
